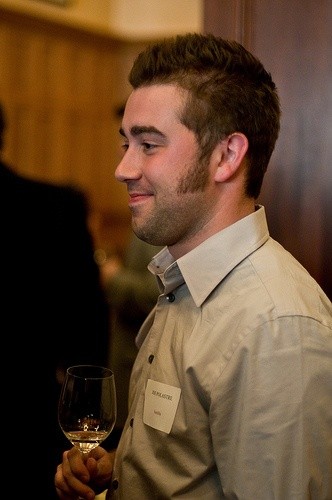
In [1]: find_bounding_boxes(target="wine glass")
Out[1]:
[58,365,116,500]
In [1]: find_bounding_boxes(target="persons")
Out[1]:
[0,103,171,500]
[53,33,332,500]
